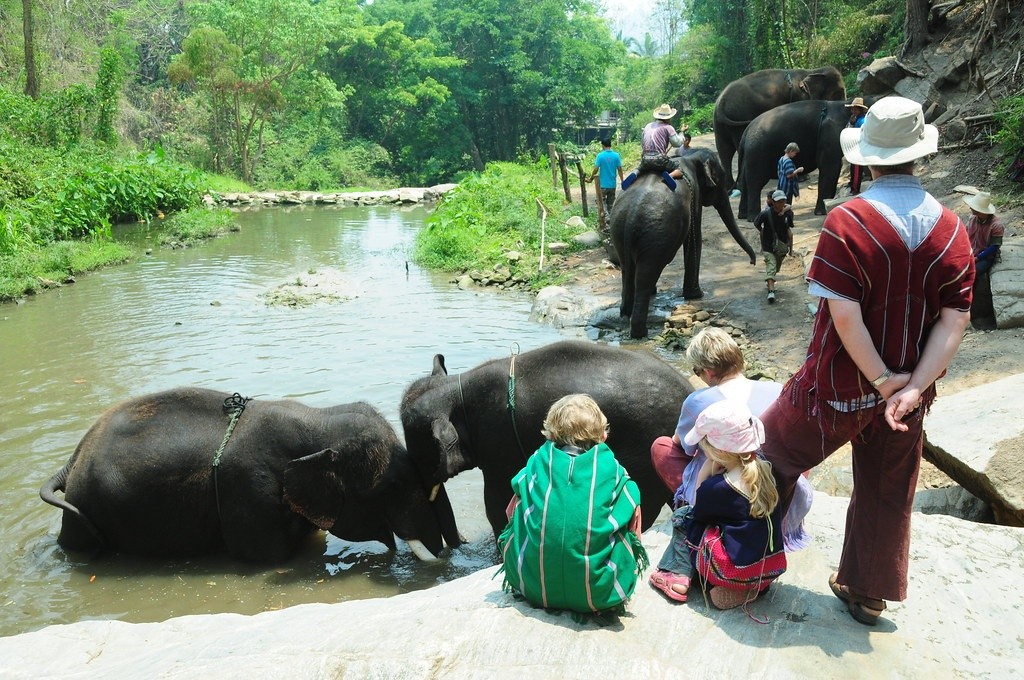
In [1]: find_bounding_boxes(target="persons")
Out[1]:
[652,325,813,547]
[622,104,688,191]
[962,191,1004,331]
[845,97,869,196]
[755,190,794,300]
[674,134,692,155]
[650,399,787,610]
[587,138,623,215]
[777,142,804,206]
[497,393,649,615]
[759,96,976,627]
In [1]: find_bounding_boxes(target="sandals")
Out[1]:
[829,572,887,626]
[710,585,757,609]
[650,569,689,601]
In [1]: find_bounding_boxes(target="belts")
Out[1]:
[642,156,663,160]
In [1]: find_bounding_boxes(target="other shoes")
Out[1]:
[844,183,850,187]
[601,225,610,232]
[663,171,676,190]
[789,251,795,258]
[966,326,994,335]
[848,192,860,196]
[621,172,638,191]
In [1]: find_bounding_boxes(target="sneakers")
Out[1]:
[767,291,775,302]
[765,281,777,290]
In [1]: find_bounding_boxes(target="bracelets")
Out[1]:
[869,369,890,386]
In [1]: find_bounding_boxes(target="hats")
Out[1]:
[845,98,868,109]
[684,400,765,454]
[839,96,938,166]
[653,104,677,120]
[772,190,787,201]
[962,192,995,214]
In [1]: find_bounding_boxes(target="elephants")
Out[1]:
[39,385,462,562]
[711,66,850,188]
[402,334,697,554]
[734,92,884,225]
[608,147,758,339]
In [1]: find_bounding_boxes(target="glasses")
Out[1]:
[693,367,711,377]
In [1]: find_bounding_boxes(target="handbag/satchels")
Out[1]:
[793,179,799,196]
[772,239,787,255]
